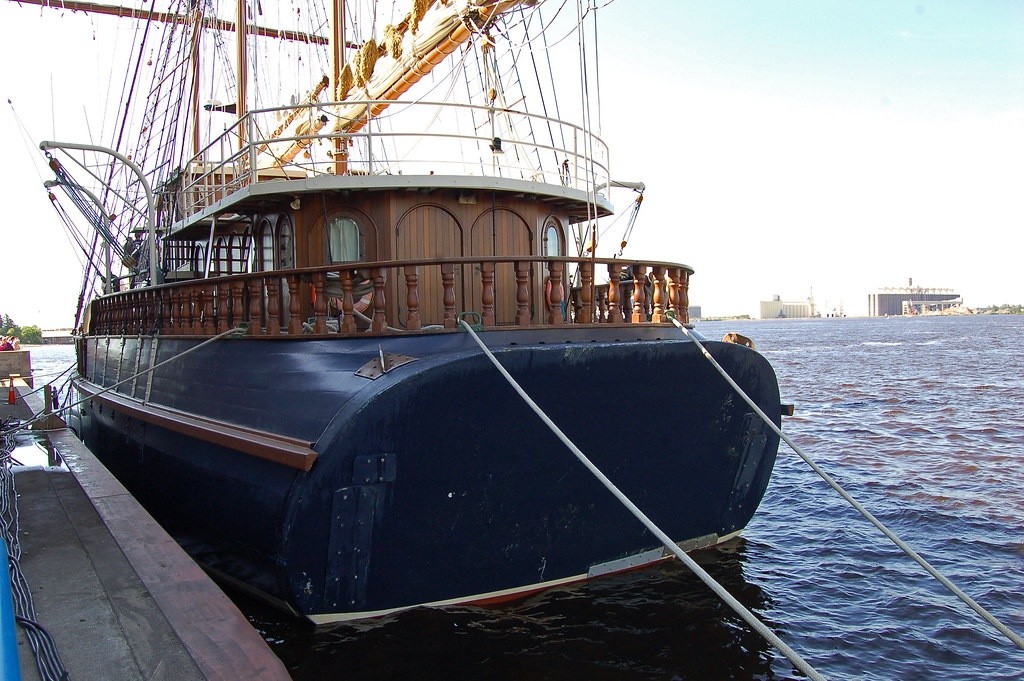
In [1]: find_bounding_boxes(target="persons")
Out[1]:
[0,336,21,350]
[0,337,15,351]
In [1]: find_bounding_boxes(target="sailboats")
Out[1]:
[0,0,794,633]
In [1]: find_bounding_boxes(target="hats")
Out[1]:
[0,336,2,339]
[8,336,15,341]
[1,338,8,343]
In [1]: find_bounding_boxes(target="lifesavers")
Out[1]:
[327,279,374,313]
[547,279,565,311]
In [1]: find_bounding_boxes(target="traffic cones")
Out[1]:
[9,377,16,404]
